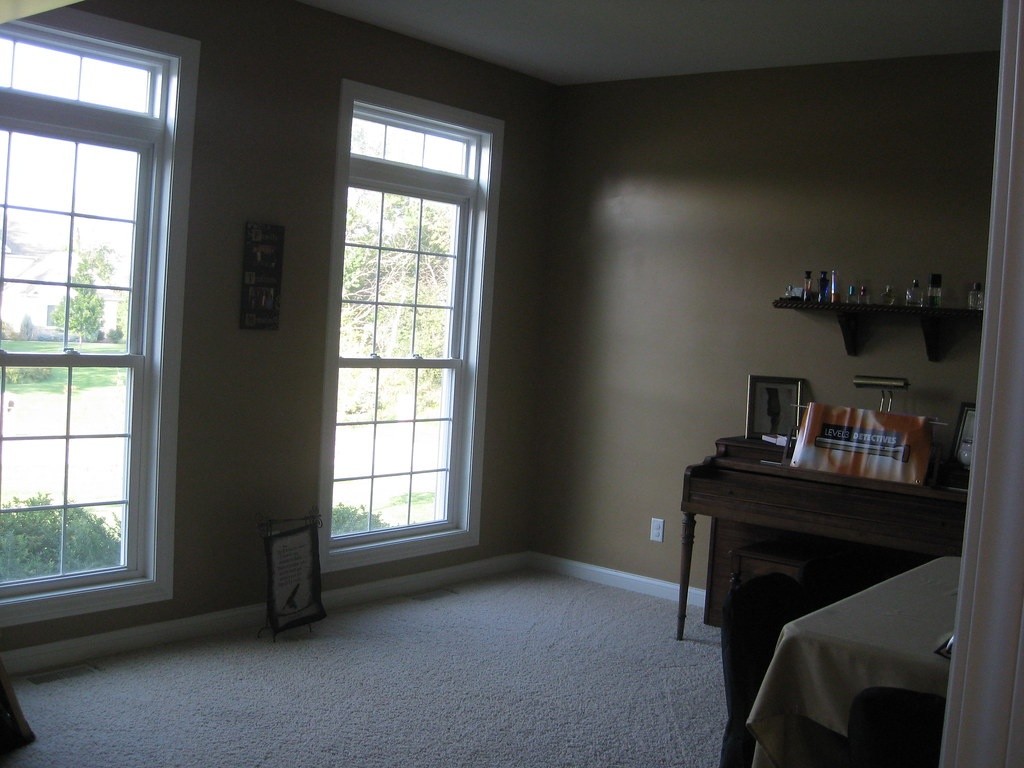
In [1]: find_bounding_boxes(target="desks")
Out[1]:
[745,555,961,768]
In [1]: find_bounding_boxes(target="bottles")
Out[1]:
[804,271,812,300]
[847,286,869,304]
[927,273,942,307]
[817,271,829,302]
[906,279,923,306]
[879,285,895,306]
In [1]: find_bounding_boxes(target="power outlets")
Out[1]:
[649,517,665,543]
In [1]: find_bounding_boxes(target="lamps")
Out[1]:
[853,375,911,413]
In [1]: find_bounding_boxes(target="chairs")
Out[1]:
[720,570,948,768]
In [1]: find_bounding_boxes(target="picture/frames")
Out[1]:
[951,401,976,477]
[745,374,803,442]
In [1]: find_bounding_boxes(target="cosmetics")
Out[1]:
[804,270,983,310]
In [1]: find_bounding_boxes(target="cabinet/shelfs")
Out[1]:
[676,435,970,642]
